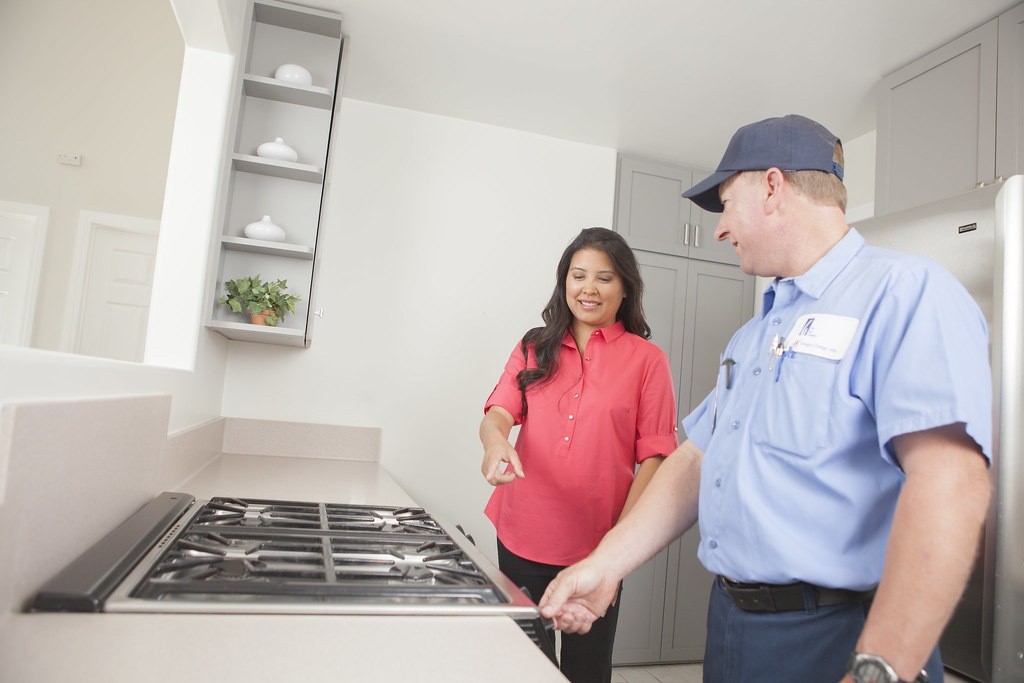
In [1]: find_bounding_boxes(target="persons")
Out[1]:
[479,227,681,683]
[538,114,993,683]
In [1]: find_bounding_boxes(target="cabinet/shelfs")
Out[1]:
[613,150,755,668]
[871,0,1024,218]
[203,0,349,350]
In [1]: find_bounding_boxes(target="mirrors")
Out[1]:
[0,0,242,383]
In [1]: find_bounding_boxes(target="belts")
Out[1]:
[719,577,876,610]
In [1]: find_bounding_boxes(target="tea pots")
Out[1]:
[273,64,313,88]
[256,138,298,163]
[243,215,286,242]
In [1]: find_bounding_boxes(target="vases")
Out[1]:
[275,64,312,86]
[244,214,286,243]
[257,137,297,163]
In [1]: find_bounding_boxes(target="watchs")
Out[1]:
[847,650,929,683]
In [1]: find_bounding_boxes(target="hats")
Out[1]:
[679,114,845,213]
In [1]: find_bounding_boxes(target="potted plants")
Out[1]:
[219,274,303,327]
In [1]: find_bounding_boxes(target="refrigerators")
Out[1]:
[845,176,1024,683]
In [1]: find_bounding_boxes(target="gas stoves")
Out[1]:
[27,489,562,681]
